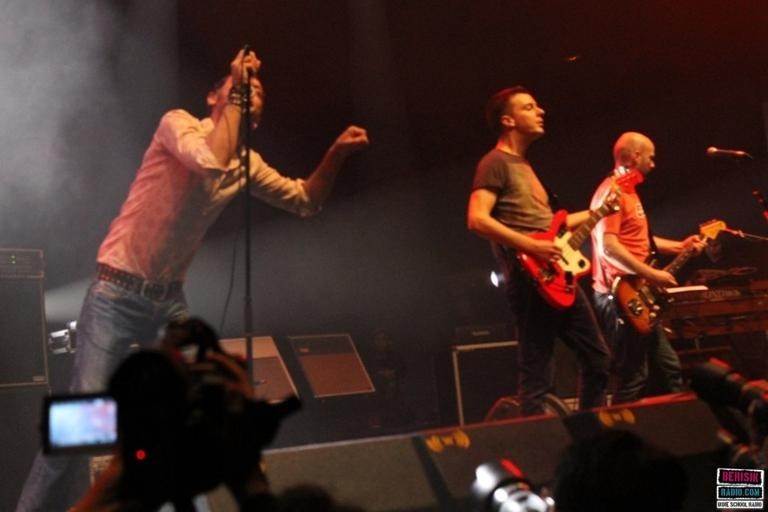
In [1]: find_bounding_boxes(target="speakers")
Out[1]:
[289,328,379,442]
[0,385,56,512]
[222,333,303,416]
[434,341,520,426]
[0,248,50,386]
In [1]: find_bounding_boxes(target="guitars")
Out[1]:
[611,217,727,334]
[514,162,645,309]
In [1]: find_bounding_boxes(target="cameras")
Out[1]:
[467,457,555,512]
[691,356,768,431]
[711,429,768,482]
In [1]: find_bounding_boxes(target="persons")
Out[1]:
[469,82,624,416]
[59,345,275,512]
[588,128,708,403]
[13,46,371,512]
[551,425,690,510]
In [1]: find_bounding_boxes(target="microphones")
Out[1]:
[705,144,755,167]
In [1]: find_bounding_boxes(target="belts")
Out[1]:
[97,263,184,301]
